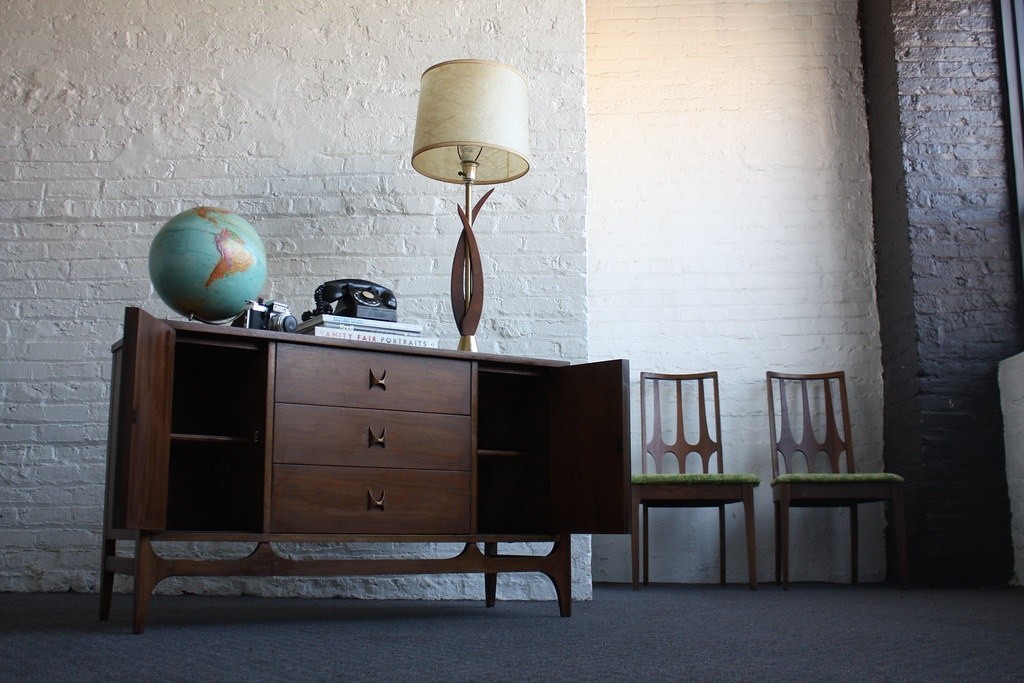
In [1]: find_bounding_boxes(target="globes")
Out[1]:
[148,205,268,325]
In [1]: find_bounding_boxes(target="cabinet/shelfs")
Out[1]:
[98,308,631,635]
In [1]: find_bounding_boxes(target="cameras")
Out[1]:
[243,295,297,332]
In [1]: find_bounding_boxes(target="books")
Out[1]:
[295,314,439,349]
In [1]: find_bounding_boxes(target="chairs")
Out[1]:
[629,371,758,591]
[766,370,911,593]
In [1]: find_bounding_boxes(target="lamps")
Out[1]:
[410,60,529,353]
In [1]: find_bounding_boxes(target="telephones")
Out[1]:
[314,278,398,322]
